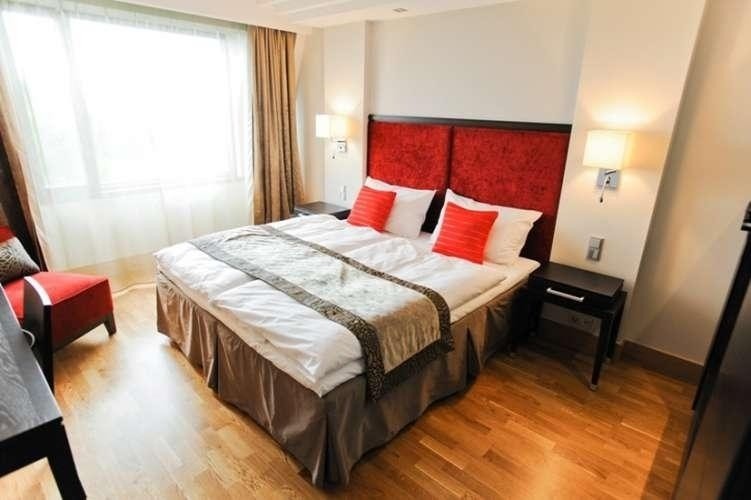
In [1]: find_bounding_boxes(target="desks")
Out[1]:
[0,283,86,500]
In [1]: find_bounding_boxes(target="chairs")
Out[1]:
[0,226,117,351]
[23,276,53,396]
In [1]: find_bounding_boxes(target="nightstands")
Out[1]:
[509,260,627,392]
[294,201,350,220]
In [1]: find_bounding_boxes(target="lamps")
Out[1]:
[315,115,359,159]
[583,129,636,203]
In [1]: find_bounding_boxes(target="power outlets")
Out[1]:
[586,236,604,260]
[340,185,345,201]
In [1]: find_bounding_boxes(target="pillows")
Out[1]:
[431,202,498,264]
[428,187,544,266]
[346,185,396,232]
[364,175,437,239]
[0,236,40,285]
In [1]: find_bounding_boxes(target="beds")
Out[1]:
[153,114,572,488]
